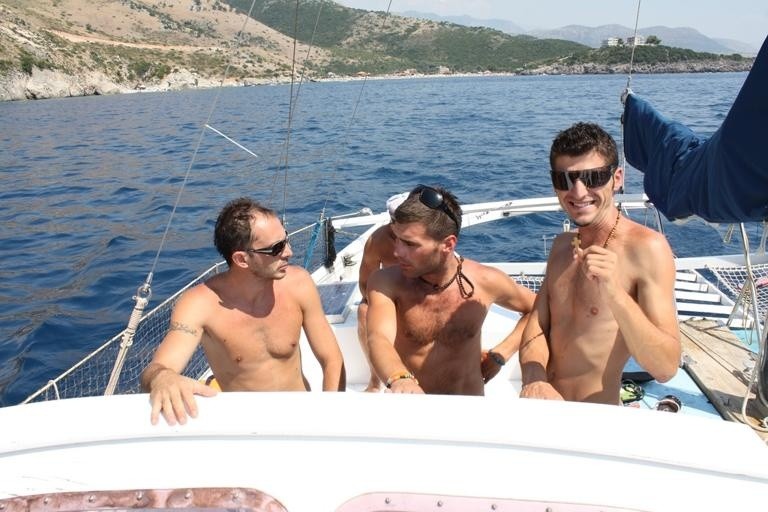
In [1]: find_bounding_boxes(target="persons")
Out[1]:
[520,122,682,406]
[141,198,346,425]
[356,192,410,391]
[364,182,538,397]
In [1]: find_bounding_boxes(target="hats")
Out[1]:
[385,191,410,220]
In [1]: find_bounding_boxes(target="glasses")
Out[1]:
[548,163,616,192]
[245,229,291,257]
[408,183,460,232]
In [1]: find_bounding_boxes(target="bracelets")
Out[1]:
[488,349,506,367]
[386,373,420,389]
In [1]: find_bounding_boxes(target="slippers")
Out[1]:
[656,394,683,414]
[620,379,646,403]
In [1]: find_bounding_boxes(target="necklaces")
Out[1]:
[570,206,621,259]
[417,253,475,298]
